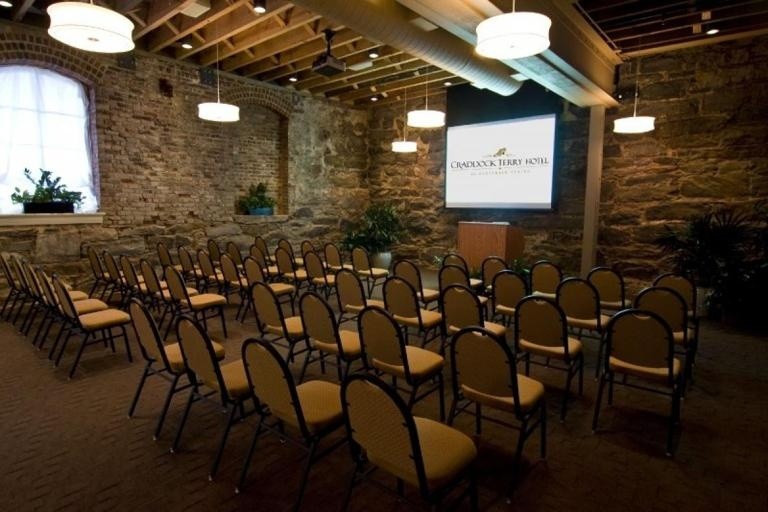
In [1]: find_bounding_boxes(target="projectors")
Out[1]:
[311,55,347,77]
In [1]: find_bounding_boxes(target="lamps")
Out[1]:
[45,1,136,54]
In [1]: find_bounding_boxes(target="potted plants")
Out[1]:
[238,179,279,216]
[654,206,759,319]
[341,205,400,270]
[10,167,88,213]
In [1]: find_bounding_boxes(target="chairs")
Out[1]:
[480,256,509,297]
[438,264,489,321]
[1,236,389,381]
[443,254,482,290]
[393,261,439,337]
[512,296,583,424]
[653,273,698,364]
[446,326,545,474]
[632,286,693,399]
[127,298,226,440]
[171,315,267,479]
[592,308,681,455]
[383,276,442,349]
[235,338,348,510]
[587,266,630,314]
[529,261,563,296]
[556,277,610,380]
[492,270,527,326]
[439,283,509,354]
[357,304,445,423]
[339,372,478,510]
